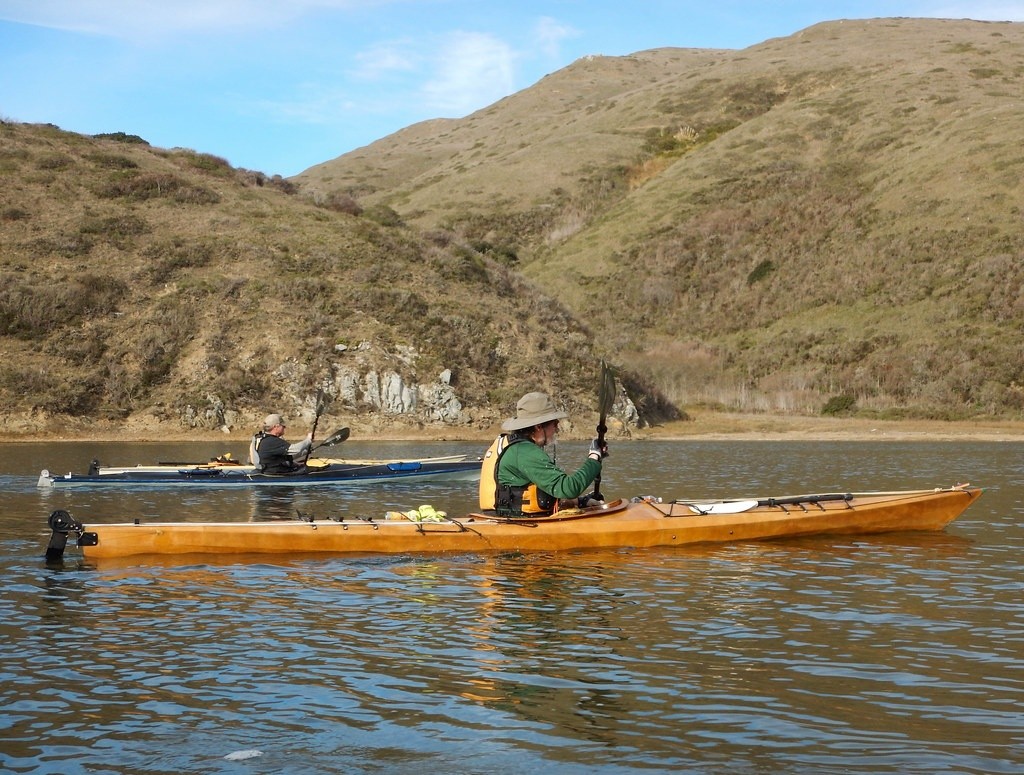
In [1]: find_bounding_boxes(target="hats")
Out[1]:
[263,413,287,430]
[501,391,569,431]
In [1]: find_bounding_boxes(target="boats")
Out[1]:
[42,485,987,561]
[37,454,484,489]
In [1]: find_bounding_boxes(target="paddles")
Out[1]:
[304,404,324,465]
[295,427,350,457]
[689,492,853,514]
[593,357,616,497]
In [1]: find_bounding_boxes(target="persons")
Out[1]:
[249,414,312,475]
[479,393,609,519]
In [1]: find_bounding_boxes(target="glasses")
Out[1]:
[551,419,559,427]
[279,423,286,428]
